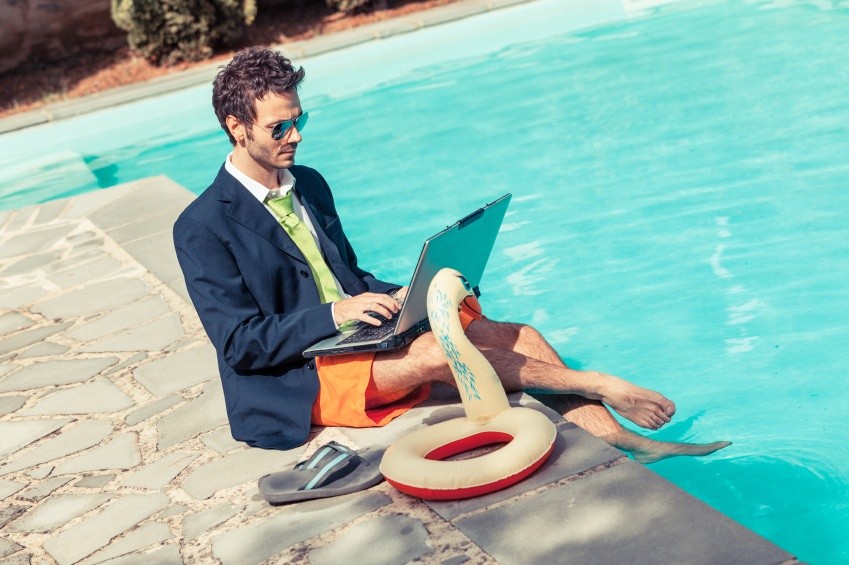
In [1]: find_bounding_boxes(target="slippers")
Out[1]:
[259,440,389,502]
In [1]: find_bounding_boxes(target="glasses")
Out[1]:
[237,112,310,141]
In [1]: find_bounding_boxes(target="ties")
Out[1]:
[266,188,361,333]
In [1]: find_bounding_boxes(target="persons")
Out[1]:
[173,46,733,465]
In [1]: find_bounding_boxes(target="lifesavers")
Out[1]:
[379,266,558,501]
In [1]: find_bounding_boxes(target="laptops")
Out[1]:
[301,194,512,356]
